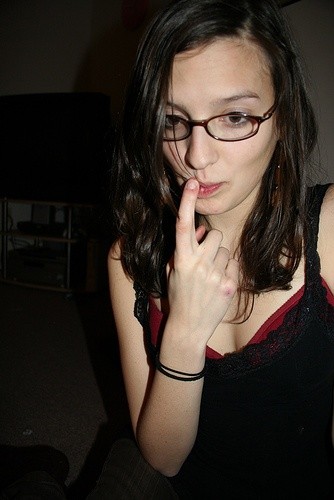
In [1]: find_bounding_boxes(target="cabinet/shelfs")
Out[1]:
[0,191,111,300]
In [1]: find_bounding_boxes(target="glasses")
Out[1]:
[163,91,280,143]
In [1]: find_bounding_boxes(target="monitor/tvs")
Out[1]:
[0,93,114,204]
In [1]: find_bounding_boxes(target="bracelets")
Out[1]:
[156,360,204,382]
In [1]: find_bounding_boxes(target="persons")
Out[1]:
[108,1,334,500]
